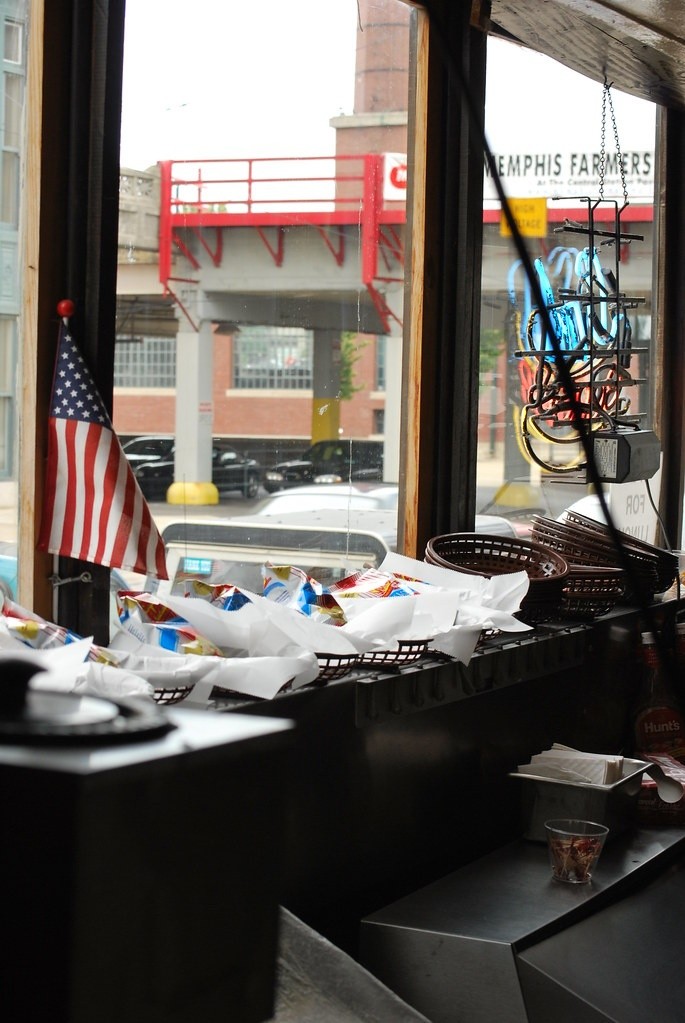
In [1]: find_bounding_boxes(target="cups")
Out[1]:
[544,819,611,884]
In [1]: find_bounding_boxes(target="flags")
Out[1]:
[38,324,169,582]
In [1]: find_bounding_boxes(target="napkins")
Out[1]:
[517,742,624,785]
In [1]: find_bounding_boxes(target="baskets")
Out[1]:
[142,507,680,706]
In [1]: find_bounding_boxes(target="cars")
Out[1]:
[151,482,548,602]
[263,438,385,494]
[119,436,261,503]
